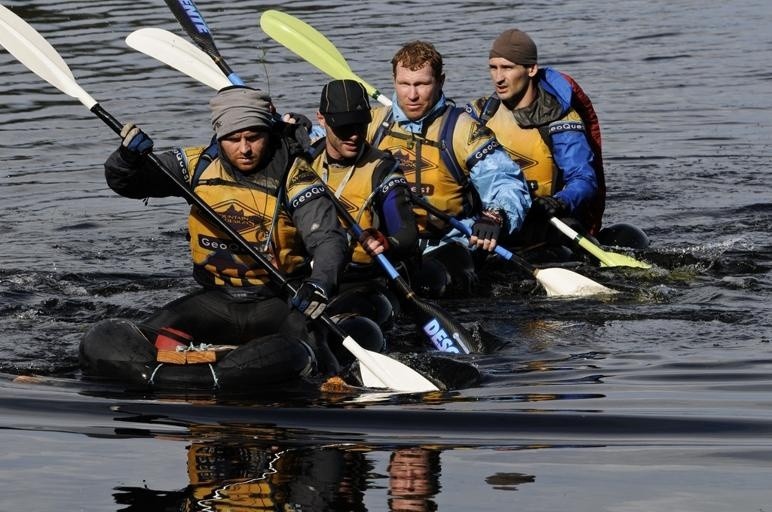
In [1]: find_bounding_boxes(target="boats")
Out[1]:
[73,205,651,404]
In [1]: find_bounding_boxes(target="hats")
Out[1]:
[319,80,372,128]
[490,29,538,66]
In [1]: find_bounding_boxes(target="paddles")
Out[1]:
[164,0,475,354]
[0,3,439,393]
[124,28,618,298]
[260,10,650,268]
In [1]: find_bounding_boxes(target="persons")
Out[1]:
[77,84,346,399]
[467,27,601,259]
[305,75,421,314]
[113,429,369,511]
[388,450,443,510]
[281,38,535,296]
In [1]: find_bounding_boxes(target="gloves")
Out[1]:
[533,195,565,218]
[294,280,328,320]
[120,121,152,165]
